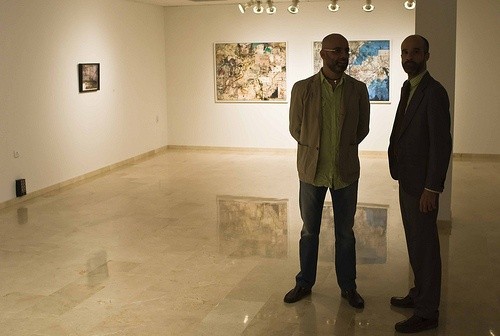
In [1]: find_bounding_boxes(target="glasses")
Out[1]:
[322,47,352,54]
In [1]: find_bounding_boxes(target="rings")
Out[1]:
[428,208,432,211]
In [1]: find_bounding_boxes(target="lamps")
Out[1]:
[265,0,277,14]
[327,0,340,12]
[253,1,264,14]
[362,0,375,13]
[287,0,301,14]
[403,0,416,10]
[239,0,255,14]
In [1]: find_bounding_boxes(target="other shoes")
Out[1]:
[341,288,364,308]
[284,285,312,304]
[395,310,439,333]
[390,293,416,308]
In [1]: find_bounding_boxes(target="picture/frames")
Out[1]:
[85,248,108,287]
[213,41,289,104]
[317,200,390,262]
[311,39,393,104]
[214,192,289,261]
[77,62,102,93]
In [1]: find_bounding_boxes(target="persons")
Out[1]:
[284,33,370,309]
[388,35,452,336]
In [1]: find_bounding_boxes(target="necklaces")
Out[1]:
[324,74,341,84]
